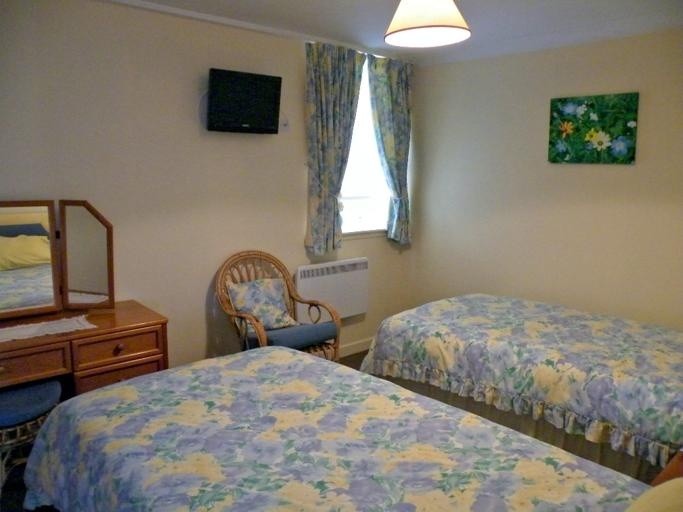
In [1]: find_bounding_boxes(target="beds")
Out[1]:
[355,292,683,484]
[20,346,683,512]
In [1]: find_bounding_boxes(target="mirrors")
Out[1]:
[61,205,115,309]
[1,200,61,317]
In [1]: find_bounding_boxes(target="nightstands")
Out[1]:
[650,450,683,486]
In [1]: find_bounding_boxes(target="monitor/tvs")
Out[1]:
[206,68,282,135]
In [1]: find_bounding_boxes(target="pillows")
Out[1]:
[225,278,299,332]
[0,207,53,234]
[1,235,52,270]
[622,475,683,511]
[2,225,47,236]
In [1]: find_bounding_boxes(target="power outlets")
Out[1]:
[280,118,291,129]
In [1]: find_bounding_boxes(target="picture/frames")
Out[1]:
[547,93,639,165]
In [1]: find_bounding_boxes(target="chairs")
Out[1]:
[214,250,342,364]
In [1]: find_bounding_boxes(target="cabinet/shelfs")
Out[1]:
[1,299,169,393]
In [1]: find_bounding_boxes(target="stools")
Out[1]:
[0,379,68,487]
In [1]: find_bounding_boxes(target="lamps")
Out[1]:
[383,0,472,48]
